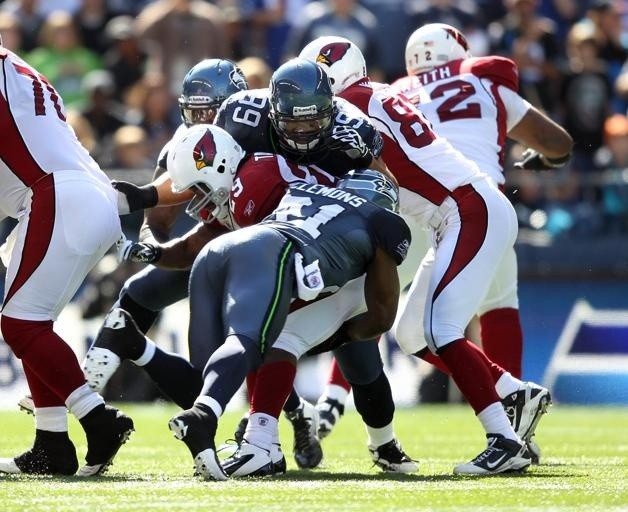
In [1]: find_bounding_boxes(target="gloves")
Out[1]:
[322,125,372,170]
[110,179,158,215]
[128,242,163,263]
[514,147,570,169]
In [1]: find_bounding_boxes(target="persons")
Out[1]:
[1,1,628,476]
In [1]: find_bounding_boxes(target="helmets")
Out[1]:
[177,58,249,128]
[298,36,368,95]
[404,23,472,76]
[268,57,334,164]
[166,124,247,225]
